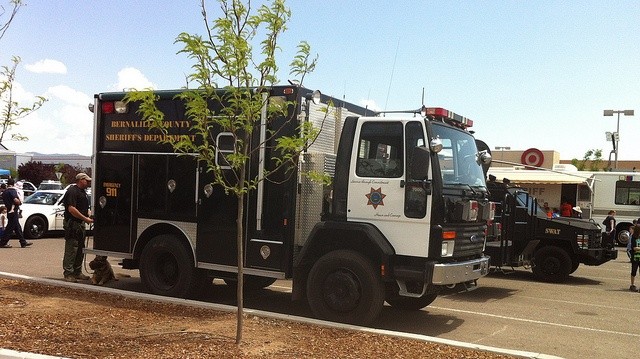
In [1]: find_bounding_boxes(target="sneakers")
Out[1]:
[8,209,15,213]
[22,242,33,248]
[65,275,78,282]
[19,210,22,214]
[76,273,90,279]
[0,244,12,248]
[630,285,636,292]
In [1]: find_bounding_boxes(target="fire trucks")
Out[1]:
[83,85,491,324]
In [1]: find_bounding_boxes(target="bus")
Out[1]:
[577,173,640,246]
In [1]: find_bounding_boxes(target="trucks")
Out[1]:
[15,181,37,199]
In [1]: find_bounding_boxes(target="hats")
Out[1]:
[14,181,23,189]
[0,183,7,191]
[76,172,92,181]
[573,206,582,213]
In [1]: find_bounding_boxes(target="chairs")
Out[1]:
[415,148,433,180]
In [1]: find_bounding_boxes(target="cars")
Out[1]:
[38,182,63,190]
[5,190,95,238]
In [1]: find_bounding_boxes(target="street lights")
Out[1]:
[604,110,634,169]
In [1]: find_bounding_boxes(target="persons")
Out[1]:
[0,184,7,206]
[561,201,572,216]
[0,179,34,248]
[552,208,560,218]
[602,210,616,244]
[545,207,552,217]
[7,181,24,218]
[0,206,7,239]
[541,202,548,213]
[572,206,583,219]
[633,218,638,225]
[63,173,95,283]
[631,218,640,292]
[626,226,635,262]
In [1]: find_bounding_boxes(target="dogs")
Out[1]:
[89,255,119,286]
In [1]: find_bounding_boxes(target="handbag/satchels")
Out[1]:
[626,249,632,260]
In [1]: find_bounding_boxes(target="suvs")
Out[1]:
[486,174,618,282]
